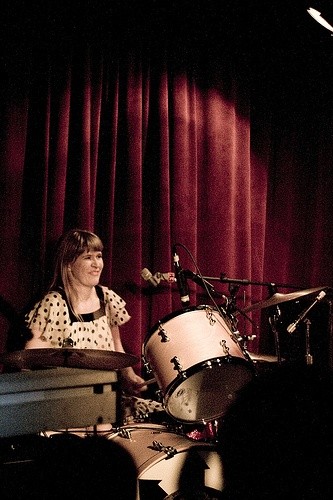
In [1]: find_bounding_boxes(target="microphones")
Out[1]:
[172,244,190,308]
[287,290,325,335]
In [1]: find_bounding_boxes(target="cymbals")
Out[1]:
[234,286,328,317]
[5,348,140,370]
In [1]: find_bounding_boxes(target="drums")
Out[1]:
[142,305,258,425]
[107,428,224,500]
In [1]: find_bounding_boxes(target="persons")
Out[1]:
[23,228,168,439]
